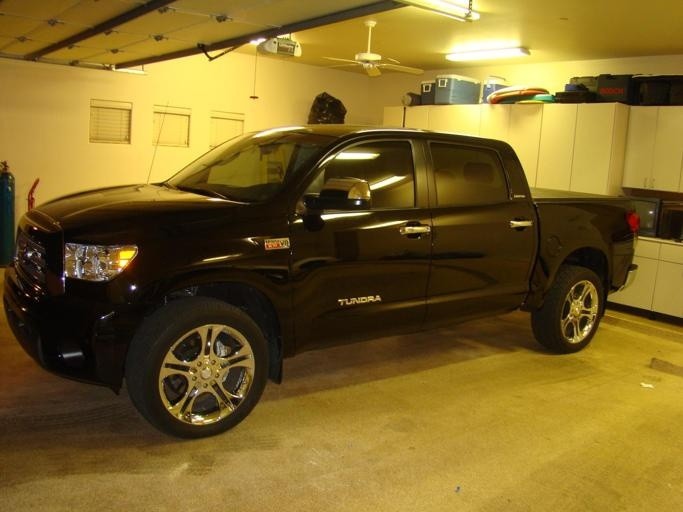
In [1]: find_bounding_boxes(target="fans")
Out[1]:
[321,20,425,76]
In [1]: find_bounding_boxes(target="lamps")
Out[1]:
[445,47,531,62]
[394,0,481,23]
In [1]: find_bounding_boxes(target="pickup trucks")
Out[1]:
[4,124,638,438]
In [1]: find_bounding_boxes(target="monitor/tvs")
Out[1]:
[629,195,662,237]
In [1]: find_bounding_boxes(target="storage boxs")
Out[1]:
[434,75,480,104]
[598,74,634,104]
[483,76,511,103]
[570,77,598,93]
[421,80,435,105]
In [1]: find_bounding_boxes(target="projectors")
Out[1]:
[256,38,302,58]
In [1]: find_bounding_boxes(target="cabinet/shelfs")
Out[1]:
[621,106,683,194]
[607,236,683,319]
[383,102,630,199]
